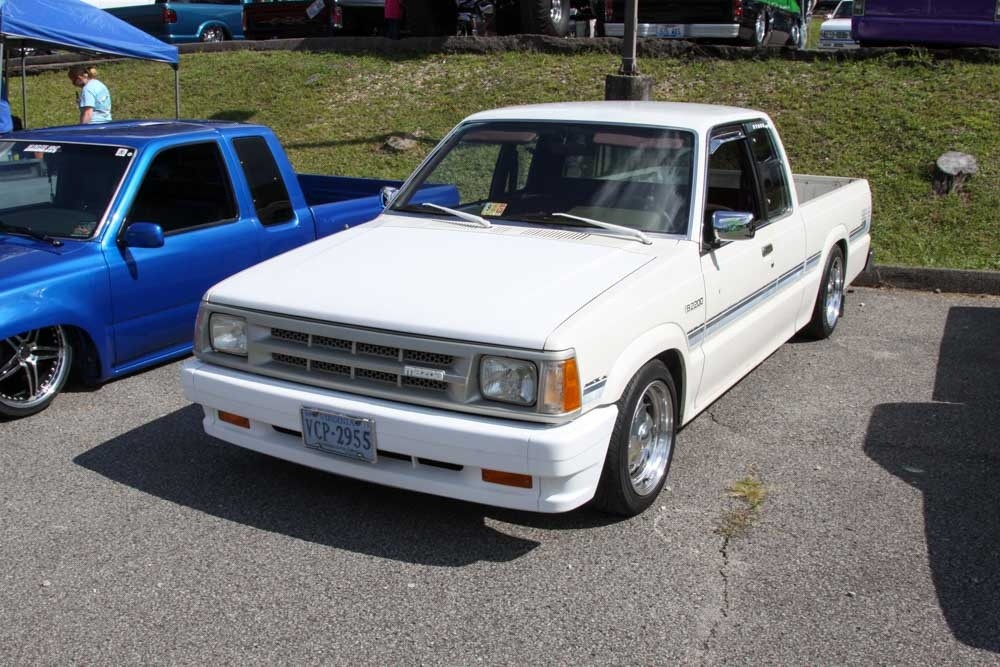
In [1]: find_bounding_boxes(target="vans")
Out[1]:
[850,0,1000,46]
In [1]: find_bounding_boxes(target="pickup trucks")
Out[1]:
[182,100,873,518]
[100,0,254,44]
[0,120,461,417]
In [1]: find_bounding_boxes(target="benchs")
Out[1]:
[534,174,754,232]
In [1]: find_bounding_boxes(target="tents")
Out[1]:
[0,0,179,129]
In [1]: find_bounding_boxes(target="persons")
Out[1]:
[0,79,13,133]
[11,116,43,159]
[68,66,112,124]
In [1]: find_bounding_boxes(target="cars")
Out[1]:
[241,0,384,40]
[817,0,861,49]
[590,0,807,48]
